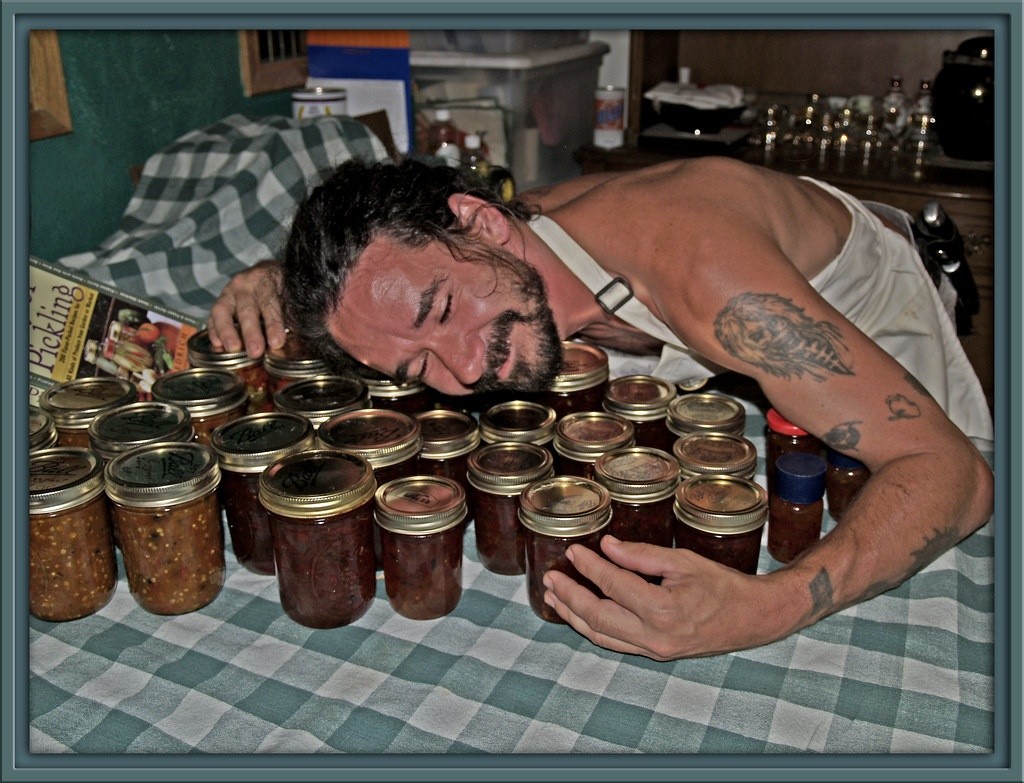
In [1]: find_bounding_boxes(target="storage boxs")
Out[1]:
[409,30,591,54]
[412,39,612,195]
[305,46,412,155]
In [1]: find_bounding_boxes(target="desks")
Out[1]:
[29,399,993,754]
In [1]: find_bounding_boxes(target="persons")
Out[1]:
[204,154,994,664]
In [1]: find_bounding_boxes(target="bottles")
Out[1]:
[459,133,488,179]
[428,111,456,156]
[24,321,874,633]
[880,74,934,133]
